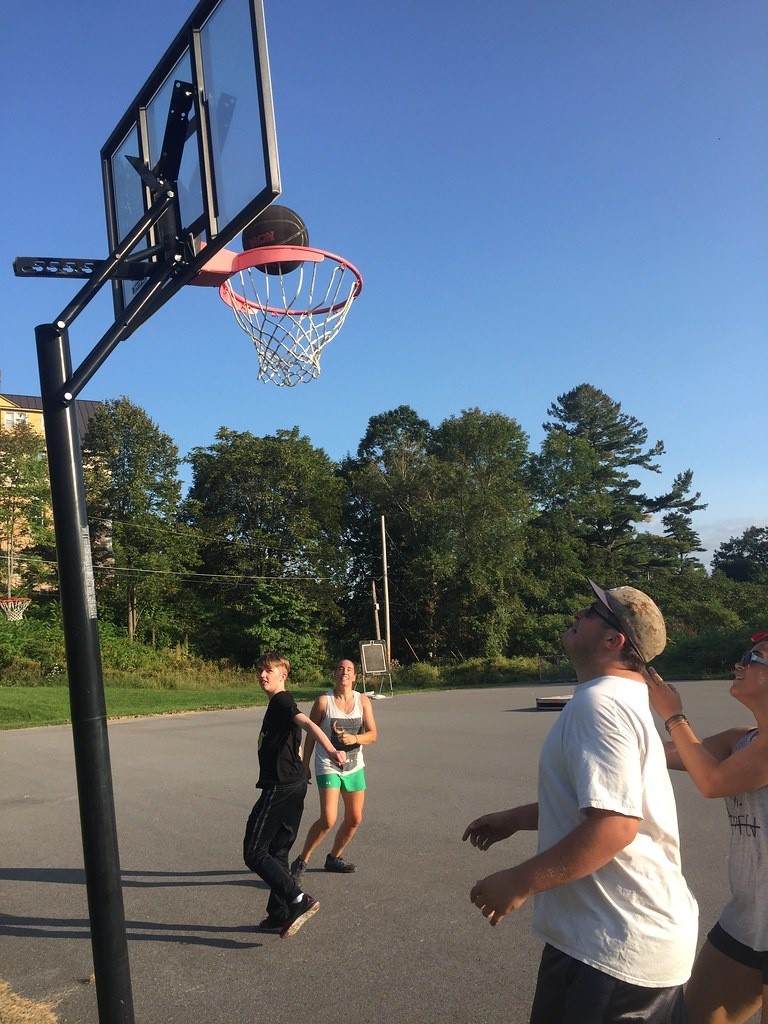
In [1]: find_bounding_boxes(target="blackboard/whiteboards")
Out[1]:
[362,640,387,675]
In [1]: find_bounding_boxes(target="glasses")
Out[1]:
[585,603,628,646]
[741,651,768,666]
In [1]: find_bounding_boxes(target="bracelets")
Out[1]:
[665,714,690,736]
[351,733,357,745]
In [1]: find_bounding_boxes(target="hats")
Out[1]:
[587,577,667,661]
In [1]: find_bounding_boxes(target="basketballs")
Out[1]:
[242,204,312,275]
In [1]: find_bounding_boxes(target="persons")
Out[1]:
[462,576,699,1024]
[643,633,768,1024]
[289,655,376,890]
[243,652,346,939]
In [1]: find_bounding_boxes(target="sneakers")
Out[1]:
[281,893,320,938]
[324,854,357,872]
[259,915,284,932]
[291,855,308,888]
